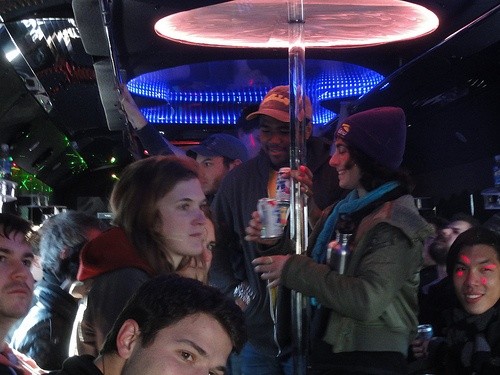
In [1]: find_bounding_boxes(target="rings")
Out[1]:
[268,257,272,261]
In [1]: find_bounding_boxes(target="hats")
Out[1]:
[337,108,406,170]
[190,133,248,161]
[246,84,313,124]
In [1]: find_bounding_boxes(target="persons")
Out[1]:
[11,212,109,370]
[417,209,500,312]
[56,140,123,225]
[245,107,432,375]
[0,212,49,375]
[118,83,248,207]
[182,205,216,283]
[409,227,500,375]
[4,223,42,342]
[68,155,208,360]
[39,275,248,375]
[207,84,353,375]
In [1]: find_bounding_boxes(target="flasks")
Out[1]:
[326,212,353,278]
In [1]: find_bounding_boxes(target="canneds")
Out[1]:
[256,198,283,238]
[414,324,432,358]
[275,166,290,206]
